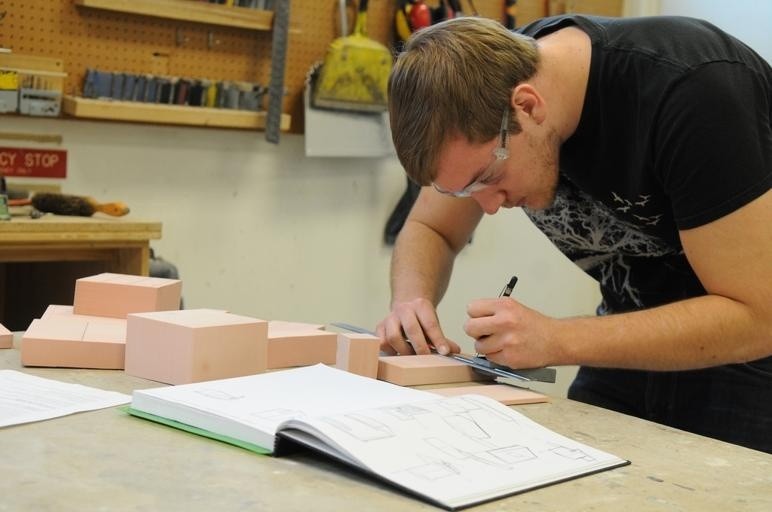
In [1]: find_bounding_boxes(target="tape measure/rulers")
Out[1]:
[269,3,283,141]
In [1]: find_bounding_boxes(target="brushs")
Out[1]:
[34,192,130,221]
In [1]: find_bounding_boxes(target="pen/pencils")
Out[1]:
[476,275,520,359]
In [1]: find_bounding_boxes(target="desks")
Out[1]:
[0,202,164,327]
[0,328,772,512]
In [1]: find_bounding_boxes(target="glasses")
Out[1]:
[429,79,530,200]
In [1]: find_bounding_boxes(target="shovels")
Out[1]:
[306,4,391,158]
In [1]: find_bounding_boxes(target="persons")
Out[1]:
[374,10,771,455]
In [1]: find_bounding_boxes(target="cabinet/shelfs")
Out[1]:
[62,0,290,134]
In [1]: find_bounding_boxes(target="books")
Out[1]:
[114,358,633,510]
